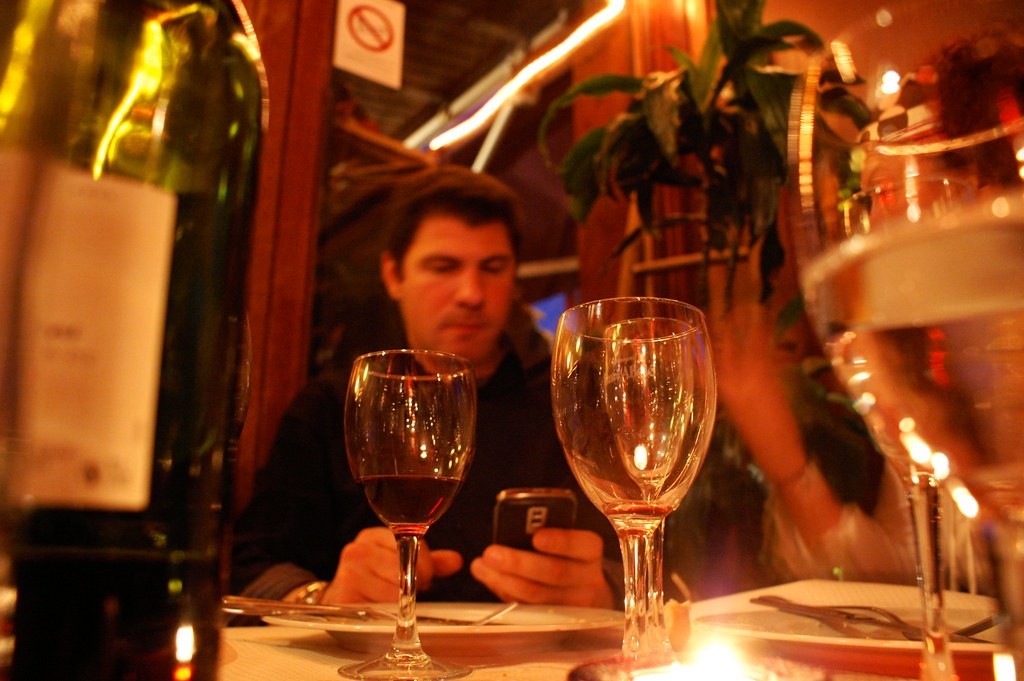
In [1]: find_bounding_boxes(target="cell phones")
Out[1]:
[493,488,577,555]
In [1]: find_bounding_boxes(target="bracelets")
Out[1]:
[295,580,327,605]
[749,460,816,497]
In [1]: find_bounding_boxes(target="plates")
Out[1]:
[260,603,627,656]
[695,608,1005,681]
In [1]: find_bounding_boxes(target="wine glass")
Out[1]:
[551,298,717,681]
[785,4,1024,681]
[337,348,475,681]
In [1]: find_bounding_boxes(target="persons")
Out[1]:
[708,305,924,588]
[228,168,661,631]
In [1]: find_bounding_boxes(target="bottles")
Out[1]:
[0,0,272,681]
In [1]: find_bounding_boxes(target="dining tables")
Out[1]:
[217,627,922,681]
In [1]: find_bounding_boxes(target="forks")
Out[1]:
[225,595,516,627]
[751,595,1012,643]
